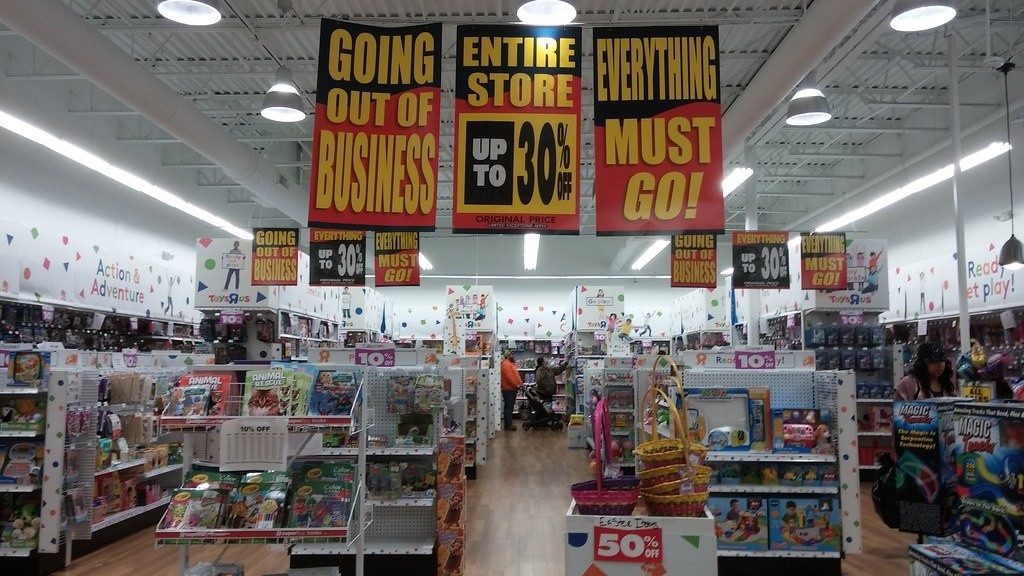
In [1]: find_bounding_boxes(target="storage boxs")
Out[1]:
[893,397,976,539]
[7,351,51,388]
[567,423,585,447]
[867,406,892,433]
[952,402,1024,563]
[908,542,1024,576]
[768,498,841,552]
[707,498,768,551]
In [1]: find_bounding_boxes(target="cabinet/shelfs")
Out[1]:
[0,231,1024,576]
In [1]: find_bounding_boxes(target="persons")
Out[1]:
[342,286,351,318]
[221,241,246,292]
[893,339,958,401]
[725,499,740,520]
[501,350,524,431]
[164,274,177,316]
[781,501,798,527]
[455,293,490,309]
[534,355,570,402]
[846,250,885,293]
[605,313,634,342]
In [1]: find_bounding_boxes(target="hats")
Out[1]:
[914,341,949,365]
[504,349,513,357]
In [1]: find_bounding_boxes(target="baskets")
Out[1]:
[571,356,712,517]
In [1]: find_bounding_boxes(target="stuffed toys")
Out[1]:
[589,439,633,475]
[10,502,40,540]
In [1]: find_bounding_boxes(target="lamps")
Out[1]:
[156,0,221,26]
[785,0,832,126]
[890,0,956,32]
[419,251,434,271]
[0,109,254,241]
[631,238,670,271]
[720,70,1015,275]
[260,0,306,123]
[995,62,1024,271]
[524,233,540,270]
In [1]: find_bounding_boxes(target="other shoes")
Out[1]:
[505,425,517,431]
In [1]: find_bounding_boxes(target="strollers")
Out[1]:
[520,384,567,432]
